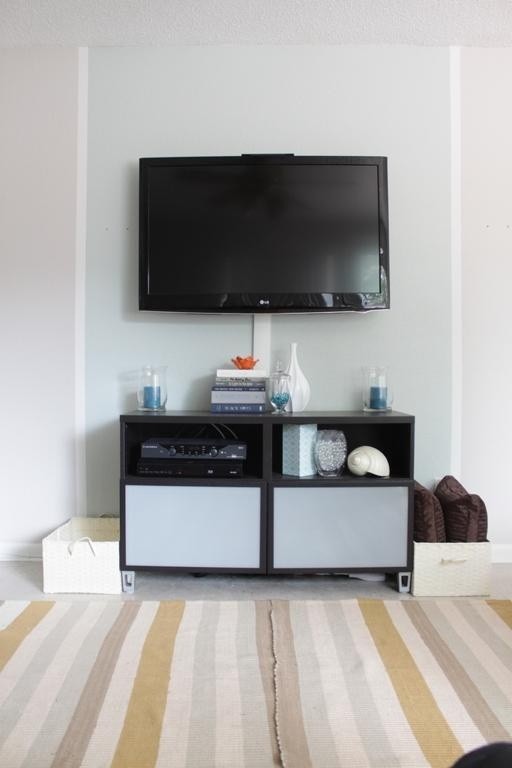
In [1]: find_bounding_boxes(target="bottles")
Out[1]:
[136,364,170,414]
[361,365,393,411]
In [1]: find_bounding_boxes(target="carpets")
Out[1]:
[271,599,511,768]
[1,599,272,767]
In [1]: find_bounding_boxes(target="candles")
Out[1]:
[370,388,387,409]
[144,386,161,408]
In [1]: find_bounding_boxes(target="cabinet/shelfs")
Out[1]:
[120,410,417,592]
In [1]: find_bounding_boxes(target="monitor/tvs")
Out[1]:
[138,153,391,314]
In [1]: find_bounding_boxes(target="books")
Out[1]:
[210,367,270,415]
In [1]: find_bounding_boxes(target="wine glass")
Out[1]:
[269,371,293,415]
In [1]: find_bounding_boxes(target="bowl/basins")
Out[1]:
[231,356,259,370]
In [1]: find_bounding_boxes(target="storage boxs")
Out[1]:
[43,516,122,593]
[413,542,492,598]
